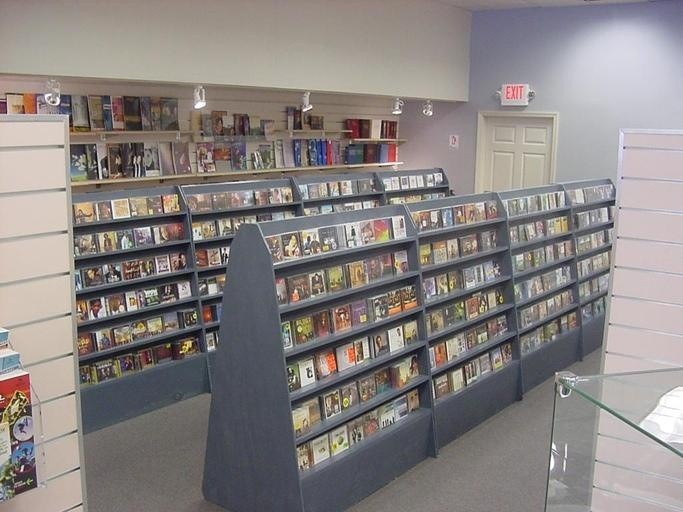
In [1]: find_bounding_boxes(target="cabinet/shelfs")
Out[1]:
[292,173,384,219]
[202,206,427,512]
[498,182,581,403]
[404,192,516,455]
[74,187,211,436]
[179,176,302,393]
[70,96,410,194]
[565,179,615,363]
[376,167,455,204]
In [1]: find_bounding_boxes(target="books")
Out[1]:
[264,215,422,471]
[0,90,193,183]
[72,192,230,389]
[188,106,397,176]
[229,172,445,234]
[410,183,619,402]
[0,328,38,501]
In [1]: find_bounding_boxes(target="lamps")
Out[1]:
[299,88,313,116]
[391,98,403,115]
[422,98,434,119]
[193,84,207,111]
[44,77,61,106]
